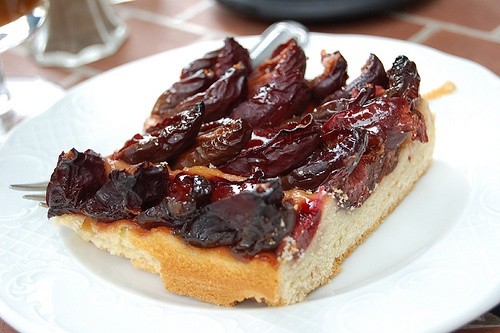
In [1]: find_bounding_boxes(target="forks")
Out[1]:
[8,20,309,209]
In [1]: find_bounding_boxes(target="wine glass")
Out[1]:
[0,59,65,140]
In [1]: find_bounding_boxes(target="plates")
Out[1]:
[0,34,500,333]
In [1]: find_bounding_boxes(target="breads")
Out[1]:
[59,92,436,308]
